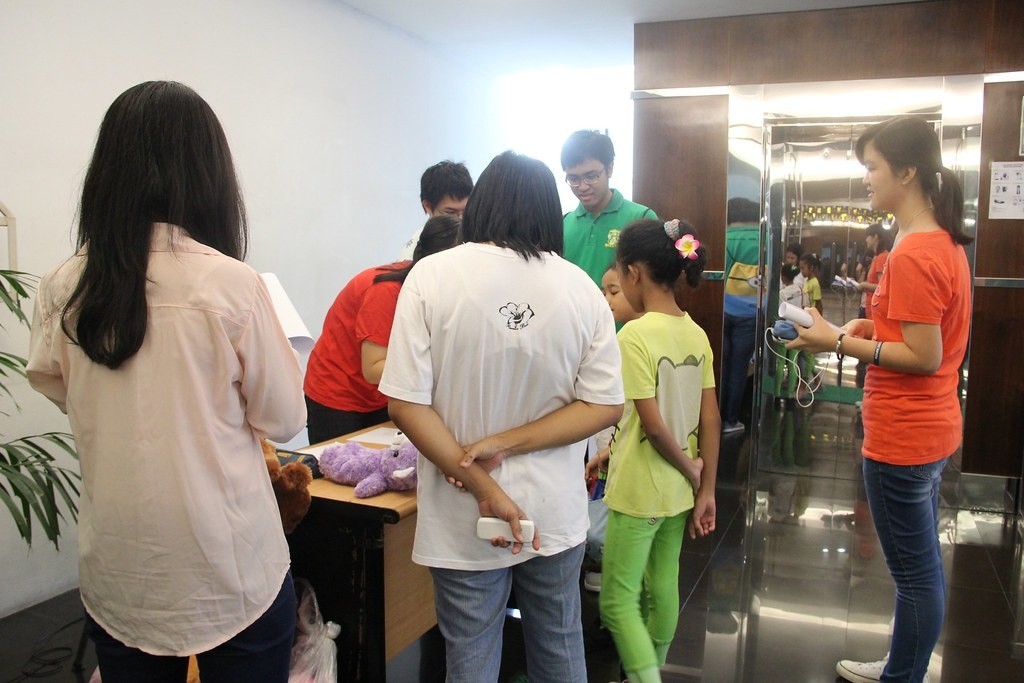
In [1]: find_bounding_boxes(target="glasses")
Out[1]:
[565,167,606,186]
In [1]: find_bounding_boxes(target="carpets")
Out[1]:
[805,383,862,405]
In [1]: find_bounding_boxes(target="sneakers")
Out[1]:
[836,649,930,683]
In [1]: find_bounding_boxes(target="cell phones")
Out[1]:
[476,518,534,543]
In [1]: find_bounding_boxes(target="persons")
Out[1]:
[305,217,460,447]
[781,243,805,308]
[395,159,474,260]
[857,246,875,318]
[721,197,760,433]
[600,219,722,683]
[840,261,847,272]
[376,149,626,683]
[775,262,803,404]
[800,253,824,391]
[785,113,973,683]
[23,80,308,683]
[856,223,889,320]
[584,262,646,591]
[559,131,660,333]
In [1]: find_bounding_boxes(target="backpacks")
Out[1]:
[725,250,767,318]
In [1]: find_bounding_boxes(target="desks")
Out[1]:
[273,420,437,683]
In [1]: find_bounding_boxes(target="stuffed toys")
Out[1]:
[318,430,417,499]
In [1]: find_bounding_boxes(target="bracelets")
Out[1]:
[837,332,845,361]
[873,341,883,366]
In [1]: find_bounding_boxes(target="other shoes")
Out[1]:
[795,389,809,399]
[809,384,824,392]
[722,420,745,432]
[584,568,601,591]
